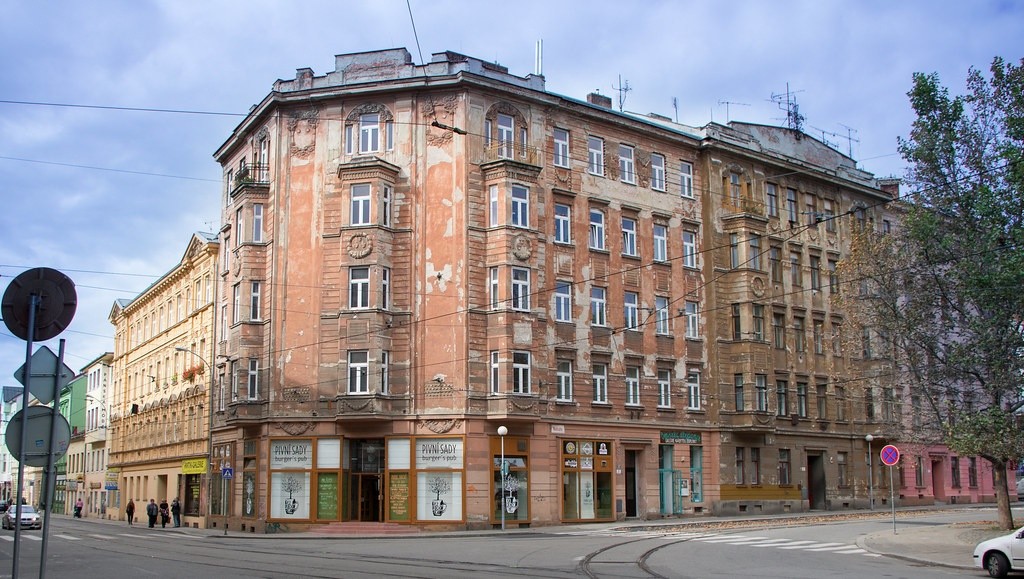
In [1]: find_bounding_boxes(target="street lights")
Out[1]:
[866,433,873,511]
[175,346,211,372]
[498,425,508,532]
[86,394,105,408]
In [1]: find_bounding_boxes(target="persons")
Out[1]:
[76,498,83,518]
[127,499,135,525]
[7,496,13,507]
[22,497,26,504]
[147,497,180,528]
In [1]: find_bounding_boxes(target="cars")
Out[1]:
[1,504,43,530]
[0,501,8,512]
[972,525,1024,578]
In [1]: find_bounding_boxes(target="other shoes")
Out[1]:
[148,525,154,528]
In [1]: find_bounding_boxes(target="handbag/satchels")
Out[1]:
[167,517,171,523]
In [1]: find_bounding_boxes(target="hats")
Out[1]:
[162,498,167,502]
